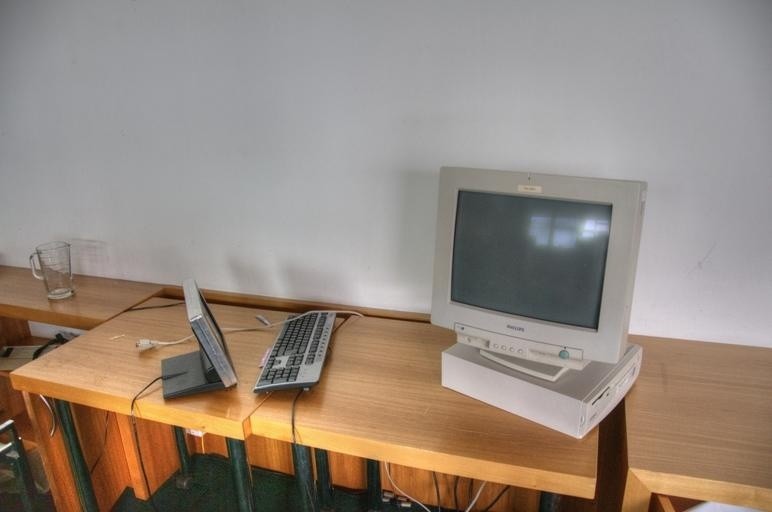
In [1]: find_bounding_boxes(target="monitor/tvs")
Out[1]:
[430,166,649,383]
[182,278,237,388]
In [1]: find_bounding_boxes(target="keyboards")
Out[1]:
[254,310,337,393]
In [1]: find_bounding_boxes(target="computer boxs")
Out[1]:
[441,336,643,440]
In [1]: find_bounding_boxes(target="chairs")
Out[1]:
[0,420,40,512]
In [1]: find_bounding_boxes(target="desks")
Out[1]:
[0,264,164,451]
[9,296,344,512]
[251,313,599,512]
[617,334,771,512]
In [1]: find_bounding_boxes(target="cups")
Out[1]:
[29,242,75,301]
